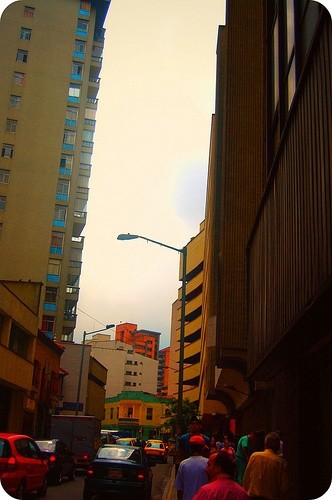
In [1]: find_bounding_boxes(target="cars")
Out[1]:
[0,432,51,500]
[30,439,75,486]
[116,438,136,446]
[83,444,156,500]
[143,439,169,464]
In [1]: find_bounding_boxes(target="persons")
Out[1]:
[175,435,210,500]
[243,432,289,500]
[193,451,252,500]
[105,434,113,444]
[173,416,256,487]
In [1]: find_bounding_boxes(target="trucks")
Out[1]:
[101,429,119,444]
[50,414,102,475]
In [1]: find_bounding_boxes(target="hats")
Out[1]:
[189,435,205,443]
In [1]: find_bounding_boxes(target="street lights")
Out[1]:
[76,324,116,415]
[117,232,187,456]
